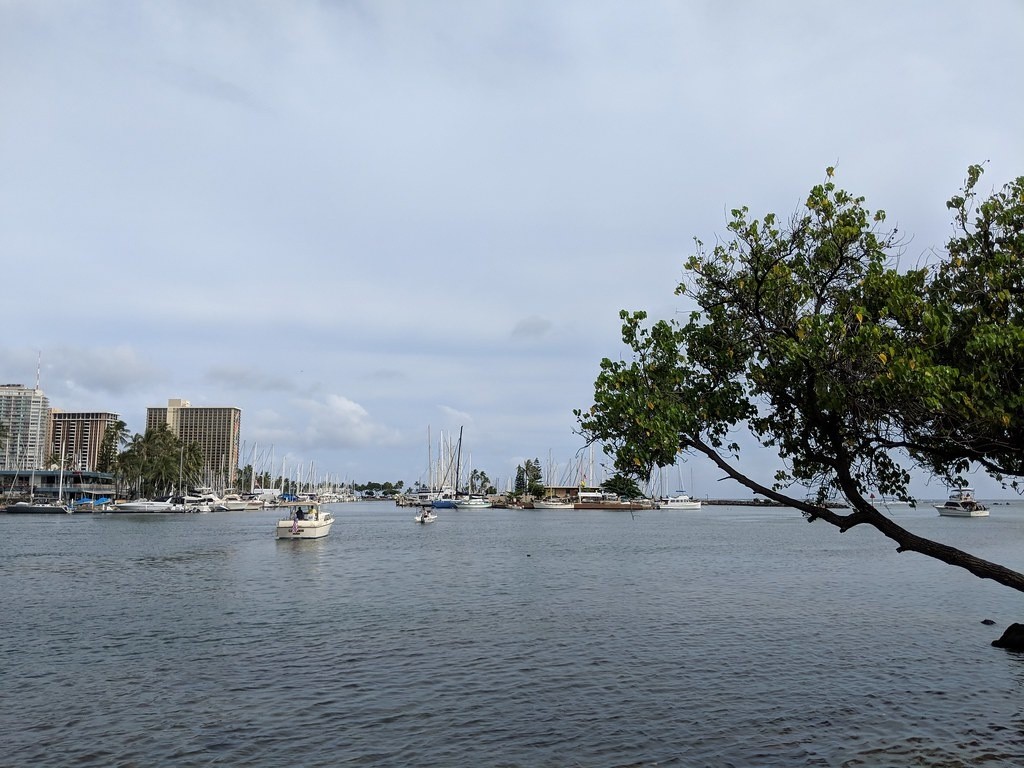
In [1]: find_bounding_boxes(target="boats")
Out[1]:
[933,488,990,517]
[413,508,439,523]
[656,459,702,511]
[275,501,336,539]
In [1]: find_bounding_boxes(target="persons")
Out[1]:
[308,506,316,517]
[296,507,304,519]
[422,506,434,518]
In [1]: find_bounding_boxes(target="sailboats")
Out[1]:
[0,440,68,514]
[396,424,493,509]
[73,439,362,513]
[485,432,655,510]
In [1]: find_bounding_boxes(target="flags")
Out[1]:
[292,514,298,533]
